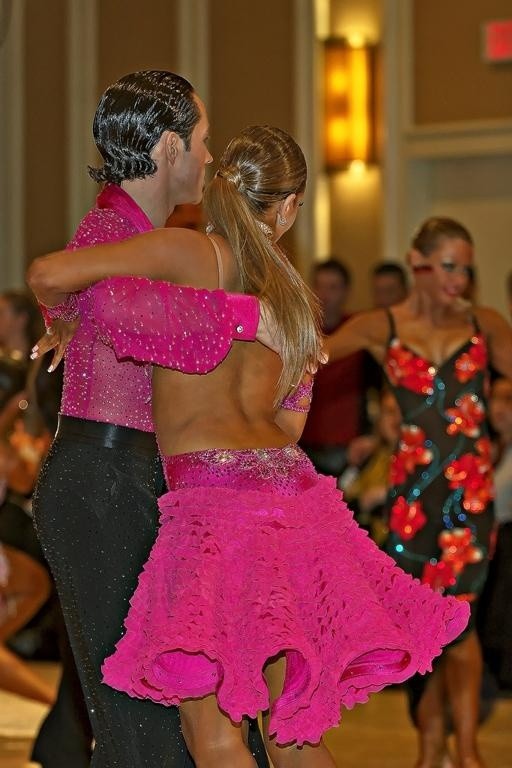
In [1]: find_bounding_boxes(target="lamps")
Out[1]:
[324,33,378,175]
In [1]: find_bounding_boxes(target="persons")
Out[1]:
[298,216,511,767]
[0,290,55,709]
[28,71,328,767]
[26,125,471,767]
